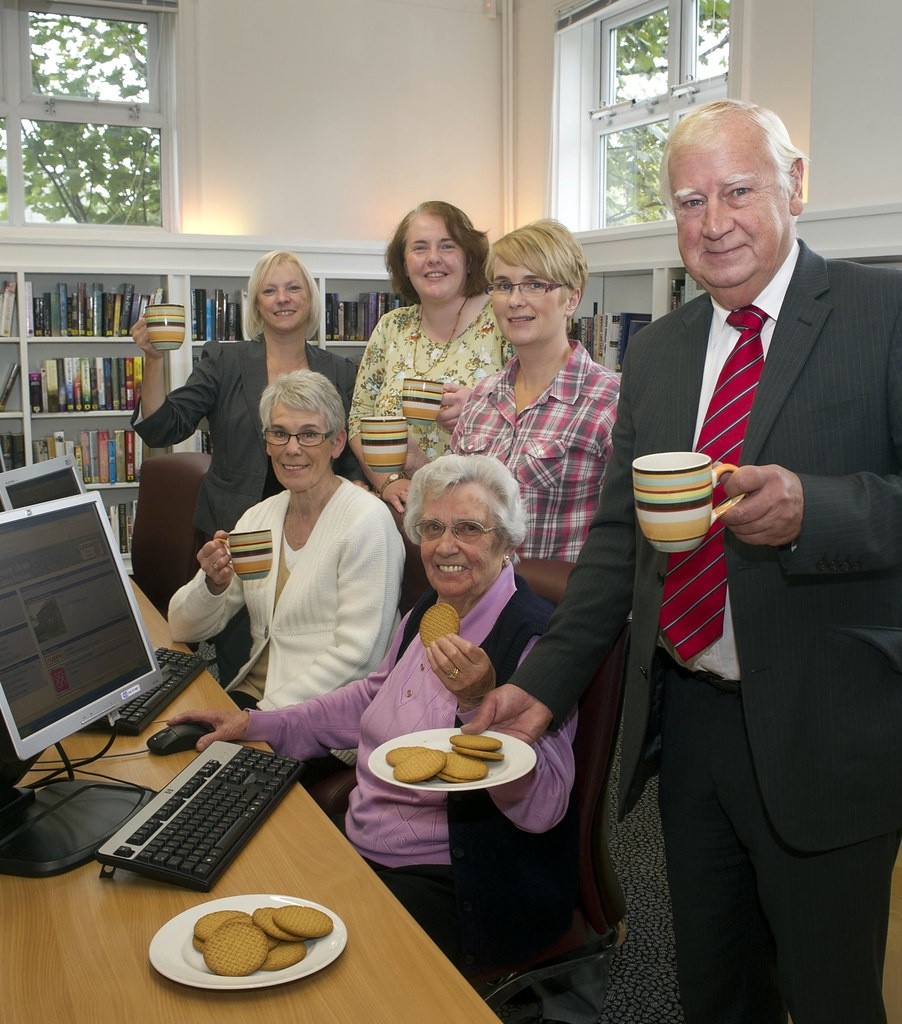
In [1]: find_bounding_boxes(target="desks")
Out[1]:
[0,573,500,1024]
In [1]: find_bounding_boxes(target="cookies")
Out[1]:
[385,734,505,783]
[192,904,333,976]
[419,602,460,647]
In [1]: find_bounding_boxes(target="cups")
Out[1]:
[215,530,273,580]
[401,379,444,426]
[360,416,408,473]
[144,304,186,351]
[632,452,746,553]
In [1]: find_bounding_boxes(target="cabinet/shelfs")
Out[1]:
[179,269,406,454]
[0,267,30,471]
[24,266,172,562]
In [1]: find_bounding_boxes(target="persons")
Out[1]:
[461,101,902,1024]
[167,370,406,782]
[450,219,621,1024]
[348,201,506,521]
[166,455,581,977]
[130,250,358,689]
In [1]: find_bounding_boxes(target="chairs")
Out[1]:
[128,455,216,611]
[397,545,627,1012]
[299,501,429,814]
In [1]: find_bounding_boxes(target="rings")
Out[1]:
[400,490,407,497]
[213,563,218,570]
[449,667,460,680]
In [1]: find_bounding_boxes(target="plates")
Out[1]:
[148,894,348,990]
[367,727,537,792]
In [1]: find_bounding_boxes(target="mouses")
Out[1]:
[146,723,210,757]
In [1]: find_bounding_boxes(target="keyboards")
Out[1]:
[92,645,210,736]
[94,741,307,893]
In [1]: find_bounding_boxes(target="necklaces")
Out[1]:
[413,296,469,376]
[287,514,308,545]
[269,357,306,376]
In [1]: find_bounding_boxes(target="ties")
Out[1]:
[656,305,770,664]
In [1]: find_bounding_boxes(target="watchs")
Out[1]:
[379,473,405,495]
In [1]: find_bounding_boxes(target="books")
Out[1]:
[568,311,652,373]
[325,290,416,342]
[0,277,164,554]
[188,287,255,455]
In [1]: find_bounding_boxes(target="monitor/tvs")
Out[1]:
[0,453,165,878]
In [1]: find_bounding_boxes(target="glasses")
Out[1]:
[261,427,335,448]
[414,518,505,544]
[485,281,568,298]
